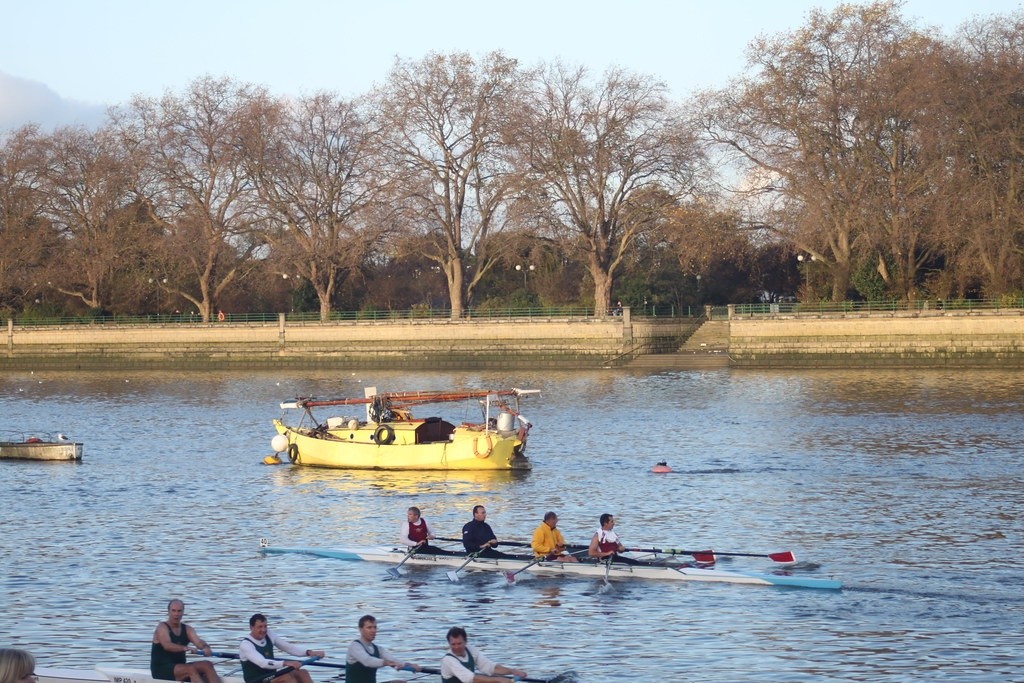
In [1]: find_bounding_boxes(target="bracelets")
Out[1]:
[306,650,310,655]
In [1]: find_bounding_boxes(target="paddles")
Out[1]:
[261,655,321,683]
[184,647,348,669]
[617,546,795,563]
[600,552,616,595]
[511,674,522,683]
[502,556,545,584]
[445,546,487,584]
[395,664,550,683]
[488,540,531,548]
[426,537,464,543]
[556,543,715,564]
[384,542,425,578]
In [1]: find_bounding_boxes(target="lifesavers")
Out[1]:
[373,424,395,445]
[286,443,298,463]
[472,434,493,459]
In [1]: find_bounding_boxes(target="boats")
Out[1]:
[0,434,84,461]
[258,538,846,592]
[261,385,543,474]
[28,665,346,683]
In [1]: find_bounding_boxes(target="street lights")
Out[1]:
[149,278,168,324]
[798,255,818,290]
[515,265,536,289]
[284,273,301,313]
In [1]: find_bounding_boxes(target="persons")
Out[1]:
[346,615,420,683]
[587,514,638,565]
[462,506,513,559]
[218,310,224,322]
[531,512,578,562]
[924,300,929,310]
[616,301,623,316]
[190,311,201,322]
[936,298,943,310]
[240,614,325,683]
[151,599,222,683]
[441,626,527,683]
[399,508,450,555]
[0,649,36,683]
[176,310,180,323]
